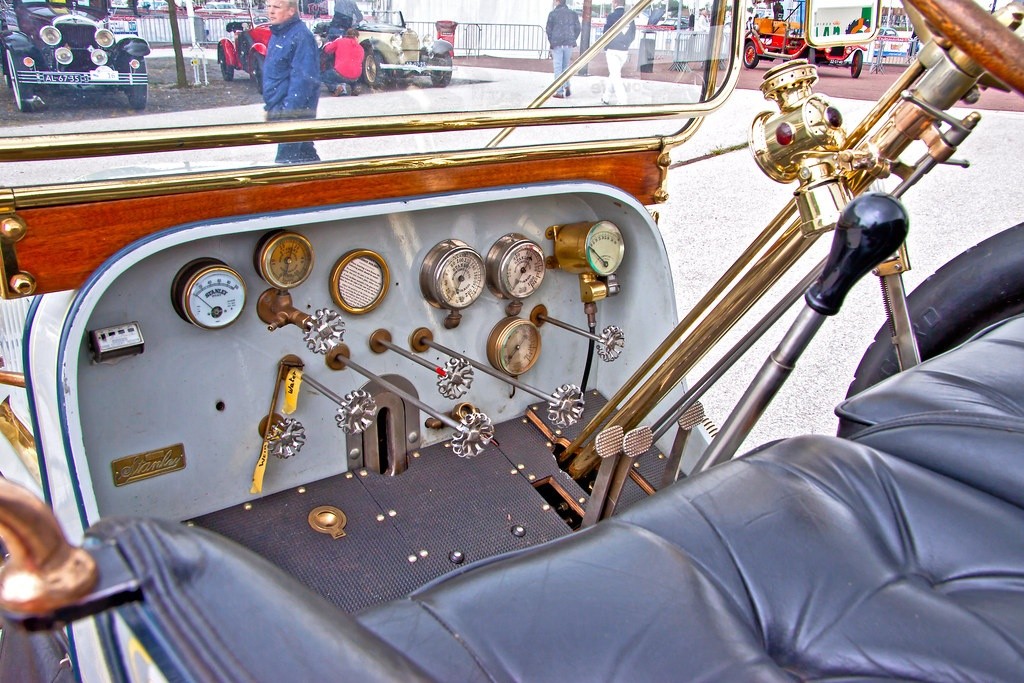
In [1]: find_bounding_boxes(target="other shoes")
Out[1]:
[335,80,353,96]
[555,86,570,99]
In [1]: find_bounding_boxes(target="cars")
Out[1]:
[314,9,455,88]
[216,15,326,96]
[744,0,869,78]
[0,0,149,113]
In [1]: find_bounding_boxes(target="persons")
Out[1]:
[696,10,709,33]
[324,29,364,97]
[325,0,362,41]
[264,0,322,164]
[602,0,636,106]
[747,16,753,30]
[546,0,581,99]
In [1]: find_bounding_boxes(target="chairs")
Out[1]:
[827,313,1023,505]
[92,433,1024,683]
